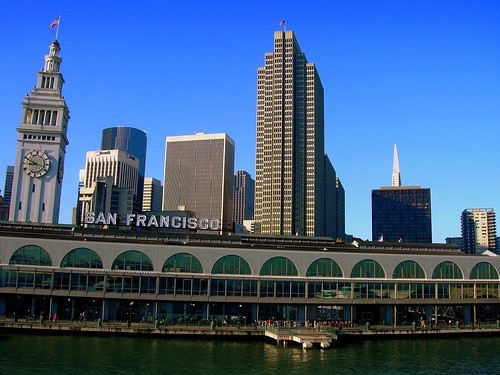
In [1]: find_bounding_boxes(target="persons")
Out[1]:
[420,317,426,330]
[40,312,87,323]
[254,316,300,328]
[304,320,351,328]
